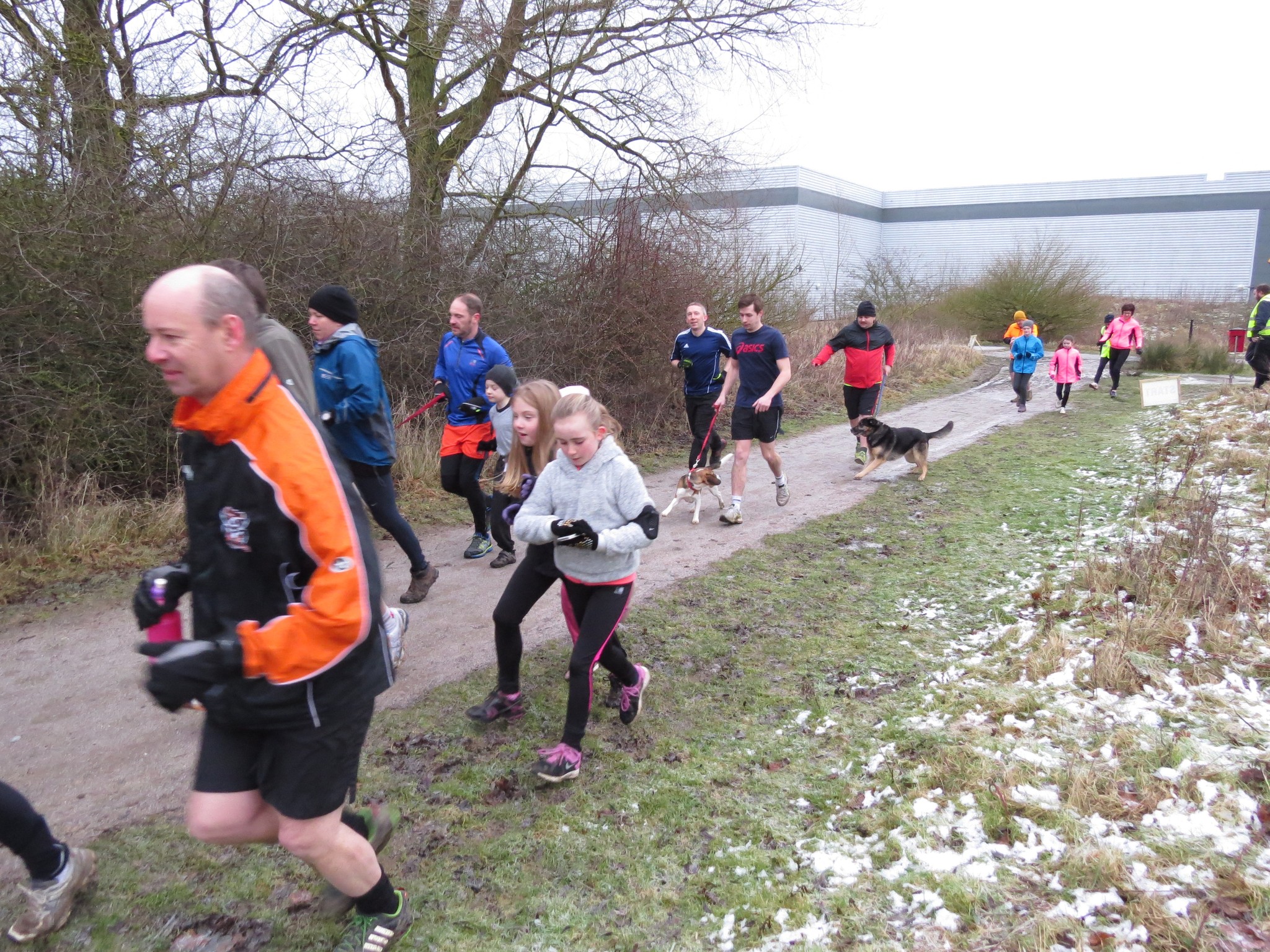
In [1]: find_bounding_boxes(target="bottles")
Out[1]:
[140,578,186,665]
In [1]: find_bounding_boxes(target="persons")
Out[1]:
[0,783,98,943]
[716,293,790,524]
[669,302,732,471]
[467,380,661,781]
[207,262,411,678]
[812,301,896,464]
[1010,319,1044,413]
[1003,311,1038,403]
[1048,334,1082,414]
[1246,284,1269,388]
[435,294,519,559]
[307,281,440,603]
[476,366,522,570]
[1088,303,1144,396]
[128,262,414,946]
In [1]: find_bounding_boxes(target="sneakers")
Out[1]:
[328,659,654,950]
[1010,382,1117,414]
[378,493,516,667]
[710,438,868,523]
[7,847,95,943]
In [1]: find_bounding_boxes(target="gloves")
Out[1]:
[133,563,195,630]
[502,504,521,525]
[1017,353,1023,359]
[433,383,452,403]
[1096,342,1105,346]
[134,637,243,713]
[551,519,597,550]
[712,370,727,384]
[520,474,537,499]
[1136,348,1143,354]
[678,358,693,369]
[1025,351,1031,358]
[459,396,485,414]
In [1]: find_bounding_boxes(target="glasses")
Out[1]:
[1123,312,1132,315]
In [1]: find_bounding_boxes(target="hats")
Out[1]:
[1022,320,1033,329]
[1104,315,1114,323]
[485,364,516,395]
[858,300,875,317]
[1014,310,1026,321]
[309,285,359,325]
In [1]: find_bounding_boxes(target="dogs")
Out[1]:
[662,453,734,524]
[850,417,953,481]
